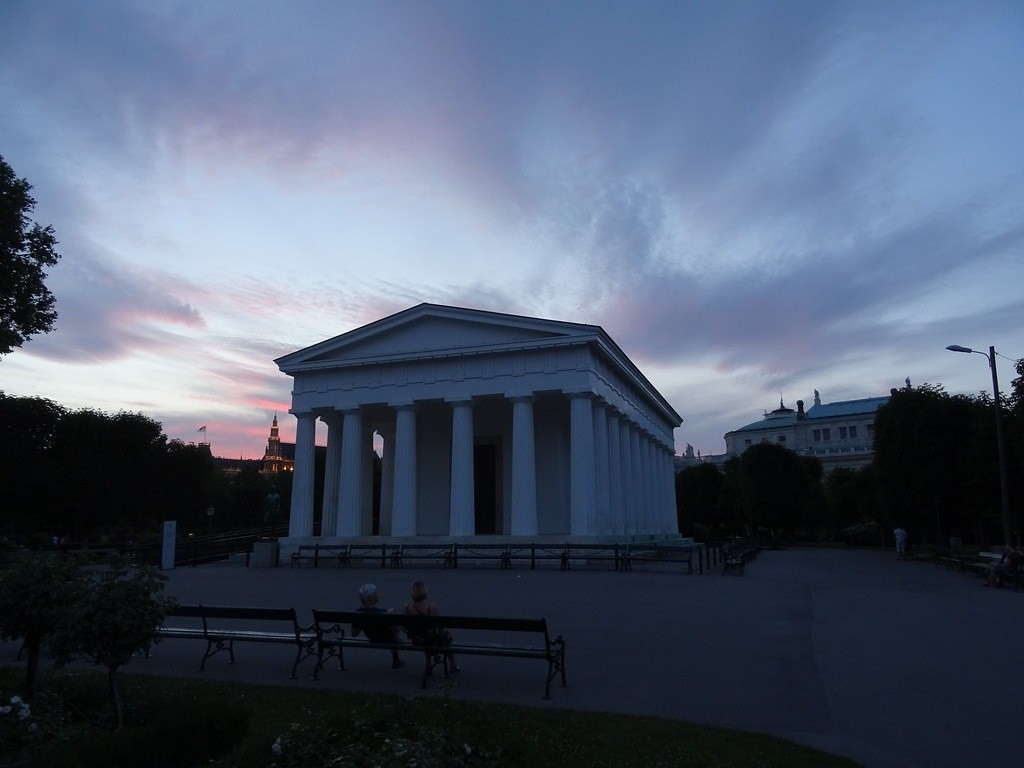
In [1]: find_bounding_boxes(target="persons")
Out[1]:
[980,539,1024,587]
[400,581,463,674]
[350,582,409,670]
[893,524,907,560]
[126,528,135,544]
[48,532,71,546]
[259,488,281,535]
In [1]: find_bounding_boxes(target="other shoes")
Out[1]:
[450,664,460,673]
[392,660,405,669]
[433,655,441,664]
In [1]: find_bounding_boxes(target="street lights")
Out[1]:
[945,344,1014,547]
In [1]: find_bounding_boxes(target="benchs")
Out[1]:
[290,544,350,569]
[156,603,324,679]
[906,542,919,561]
[310,608,568,700]
[502,544,569,571]
[623,544,695,574]
[448,543,512,570]
[930,547,1024,591]
[341,544,404,569]
[716,537,761,577]
[393,544,456,569]
[562,543,628,572]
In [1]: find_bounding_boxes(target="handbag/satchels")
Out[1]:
[438,630,453,646]
